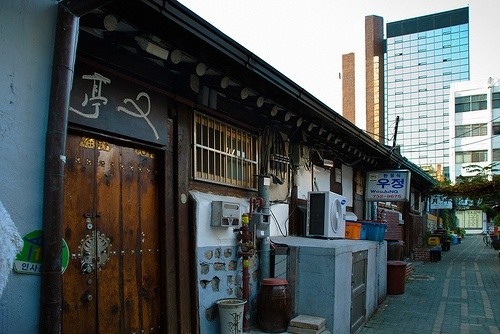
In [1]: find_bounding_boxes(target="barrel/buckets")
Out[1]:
[386,260,407,295]
[254,278,293,334]
[441,238,450,252]
[429,248,442,262]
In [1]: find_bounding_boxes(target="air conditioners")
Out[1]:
[306,190,347,239]
